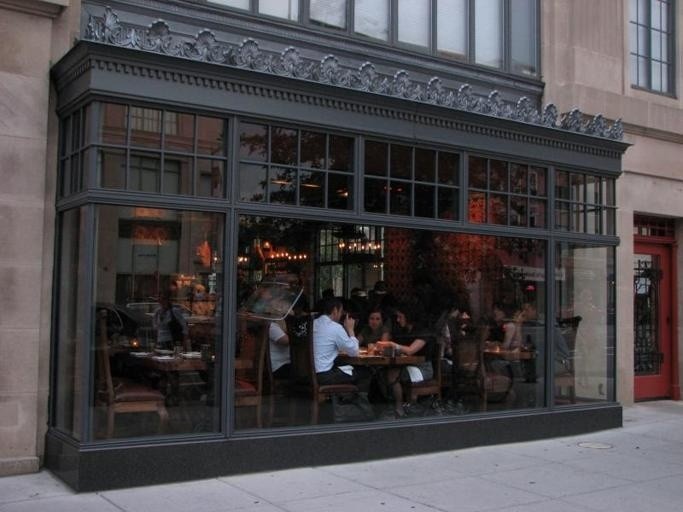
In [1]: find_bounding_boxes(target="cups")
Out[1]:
[368,343,381,356]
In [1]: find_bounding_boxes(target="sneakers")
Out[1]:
[382,396,472,420]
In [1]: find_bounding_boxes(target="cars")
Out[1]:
[95,301,160,347]
[125,299,218,349]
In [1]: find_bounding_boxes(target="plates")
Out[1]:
[132,348,200,360]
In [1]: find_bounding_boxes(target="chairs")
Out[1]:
[95,312,585,442]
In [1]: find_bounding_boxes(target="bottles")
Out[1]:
[198,334,209,361]
[173,341,184,360]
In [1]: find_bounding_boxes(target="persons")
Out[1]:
[437,301,572,414]
[151,290,188,405]
[246,280,434,417]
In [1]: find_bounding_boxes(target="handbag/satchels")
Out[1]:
[165,319,185,342]
